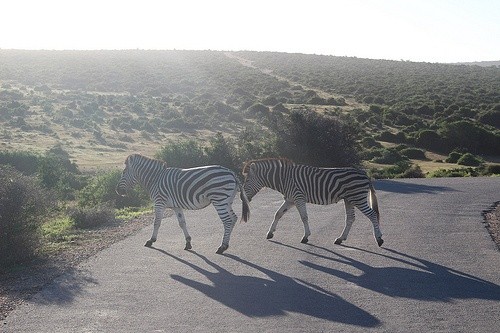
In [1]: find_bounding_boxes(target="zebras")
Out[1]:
[239,157,384,247]
[116,153,252,254]
